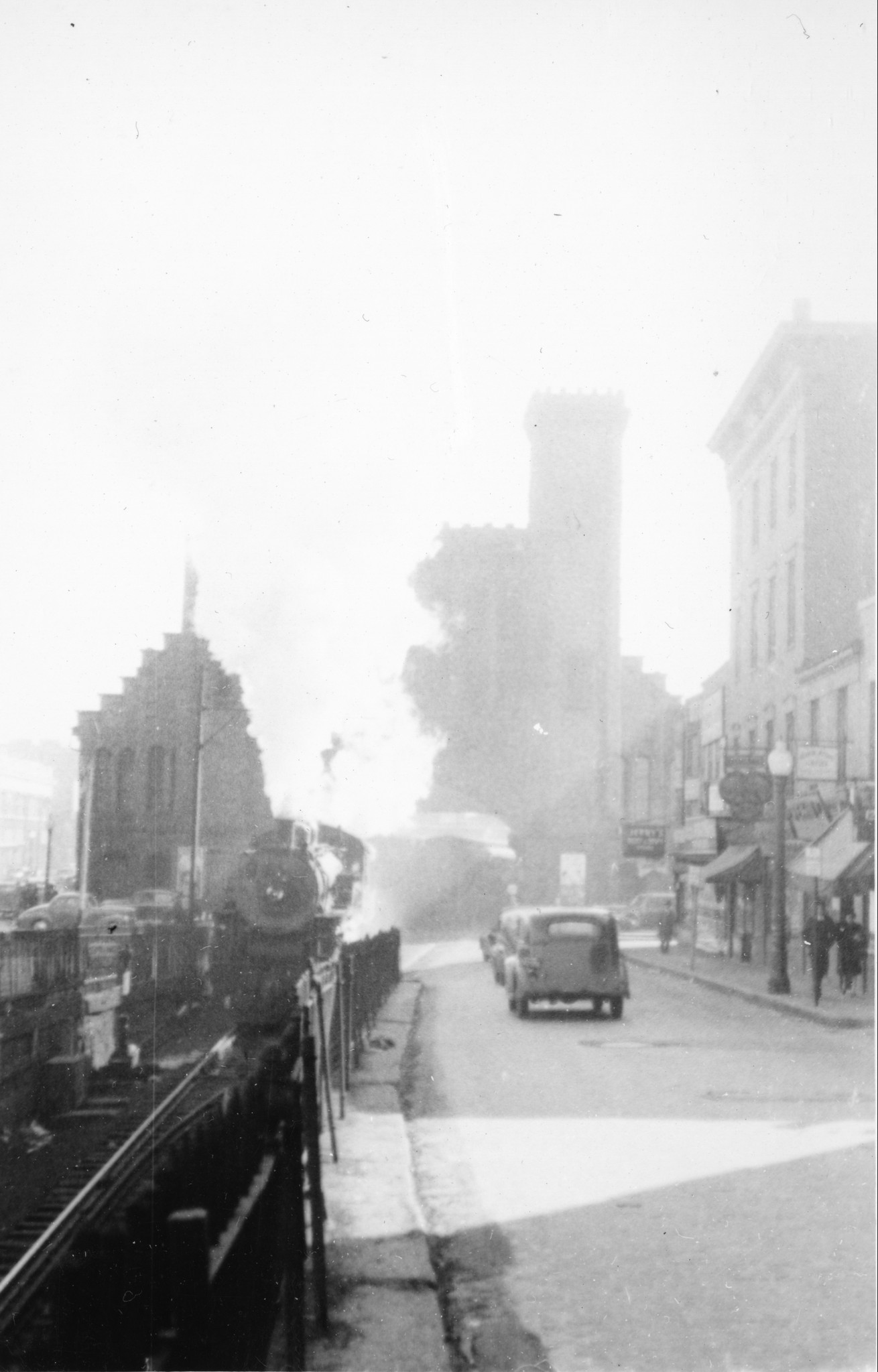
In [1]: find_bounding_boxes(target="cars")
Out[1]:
[478,906,631,1019]
[12,889,98,931]
[77,889,176,931]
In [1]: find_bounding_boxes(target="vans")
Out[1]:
[617,893,677,929]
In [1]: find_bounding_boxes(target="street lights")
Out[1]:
[768,741,794,996]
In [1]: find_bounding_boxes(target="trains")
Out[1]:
[203,814,366,1026]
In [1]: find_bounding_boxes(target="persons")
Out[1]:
[801,900,837,1007]
[833,909,868,997]
[657,899,676,954]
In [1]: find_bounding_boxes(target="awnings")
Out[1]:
[694,843,763,886]
[783,810,873,880]
